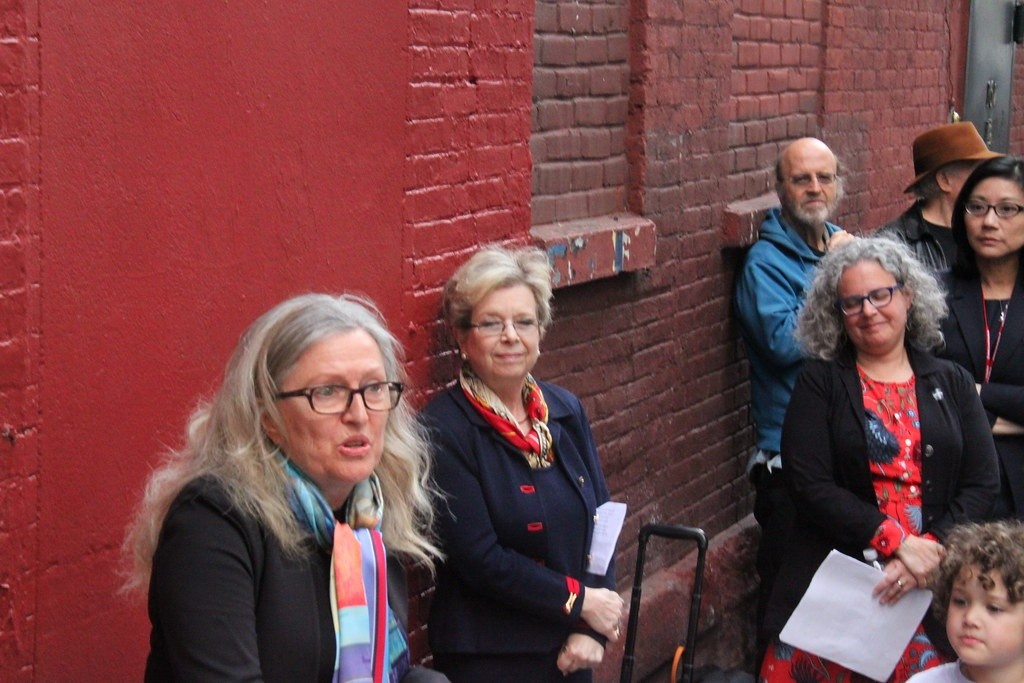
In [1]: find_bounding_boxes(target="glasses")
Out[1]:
[834,284,903,317]
[272,381,404,414]
[462,318,542,336]
[964,201,1024,217]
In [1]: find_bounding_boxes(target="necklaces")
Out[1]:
[518,410,529,424]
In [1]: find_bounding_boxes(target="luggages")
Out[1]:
[619,524,754,683]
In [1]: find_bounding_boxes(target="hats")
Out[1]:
[902,122,1006,194]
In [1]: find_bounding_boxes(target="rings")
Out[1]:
[615,628,620,634]
[897,580,903,588]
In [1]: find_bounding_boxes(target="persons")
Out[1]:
[931,153,1024,519]
[734,135,857,683]
[141,294,452,683]
[905,520,1024,683]
[414,243,630,683]
[781,234,1000,663]
[874,121,1009,302]
